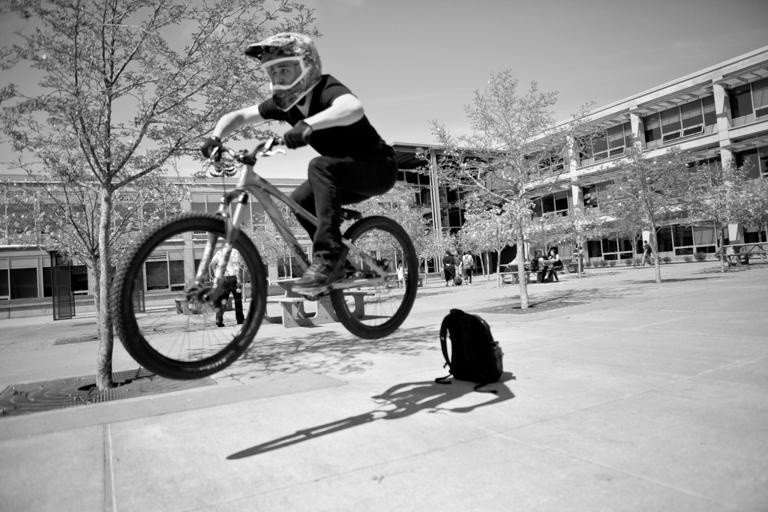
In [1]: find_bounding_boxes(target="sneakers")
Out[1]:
[293,261,347,289]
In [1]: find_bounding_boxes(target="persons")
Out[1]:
[641,240,654,267]
[443,249,476,287]
[397,260,406,289]
[530,248,562,282]
[201,32,400,288]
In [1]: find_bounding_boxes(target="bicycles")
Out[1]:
[104,128,420,383]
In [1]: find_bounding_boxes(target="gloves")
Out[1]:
[201,136,223,158]
[284,120,313,148]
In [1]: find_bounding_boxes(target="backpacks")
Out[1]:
[435,309,503,394]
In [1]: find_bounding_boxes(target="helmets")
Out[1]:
[244,32,322,112]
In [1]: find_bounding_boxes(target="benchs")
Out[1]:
[499,269,563,285]
[174,298,193,315]
[245,292,374,328]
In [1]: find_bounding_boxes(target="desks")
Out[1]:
[498,260,553,284]
[270,277,301,327]
